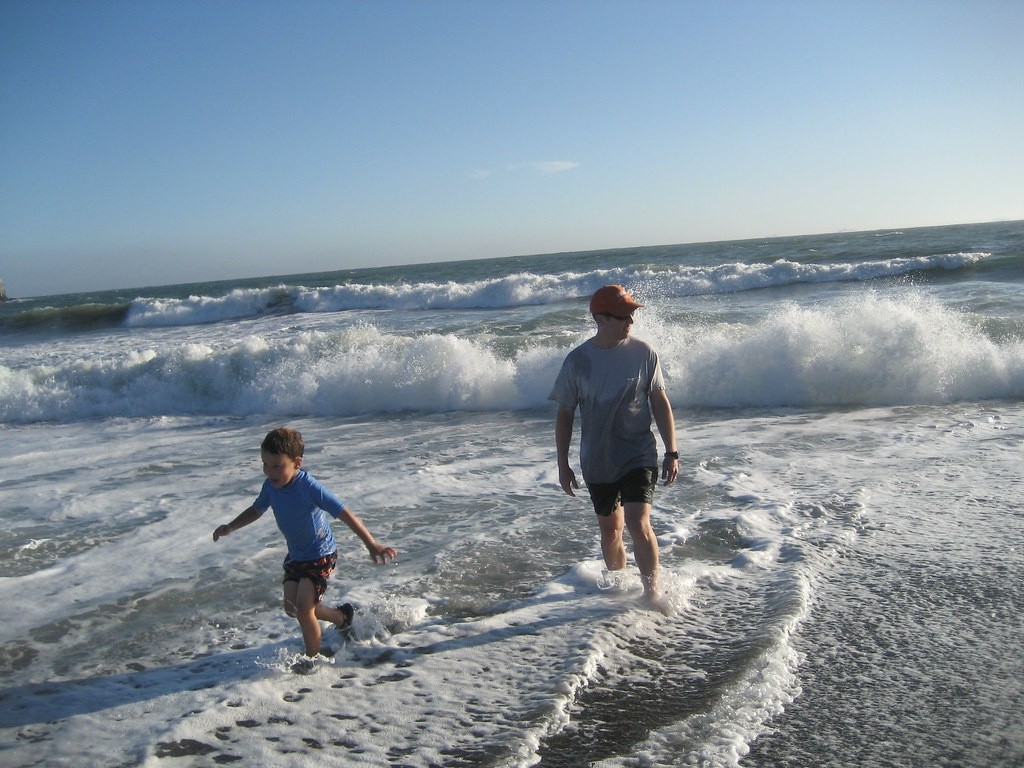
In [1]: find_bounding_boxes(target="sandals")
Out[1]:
[334,602,353,637]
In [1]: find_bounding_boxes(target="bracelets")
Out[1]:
[664,451,680,459]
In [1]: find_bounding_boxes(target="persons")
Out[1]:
[547,285,679,577]
[213,428,398,659]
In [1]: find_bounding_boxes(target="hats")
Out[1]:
[590,285,644,316]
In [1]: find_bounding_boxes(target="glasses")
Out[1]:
[604,311,634,320]
